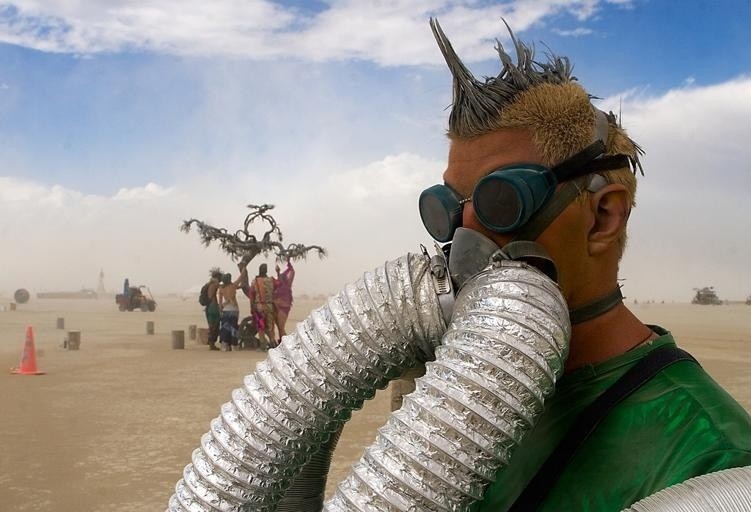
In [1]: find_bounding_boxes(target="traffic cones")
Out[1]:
[11,324,46,377]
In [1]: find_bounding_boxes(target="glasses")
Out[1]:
[419,140,629,242]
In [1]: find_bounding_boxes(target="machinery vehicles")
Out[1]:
[115,279,156,311]
[691,287,722,305]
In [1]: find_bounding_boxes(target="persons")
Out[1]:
[391,16,751,512]
[205,262,294,352]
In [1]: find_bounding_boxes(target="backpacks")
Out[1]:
[198,282,215,306]
